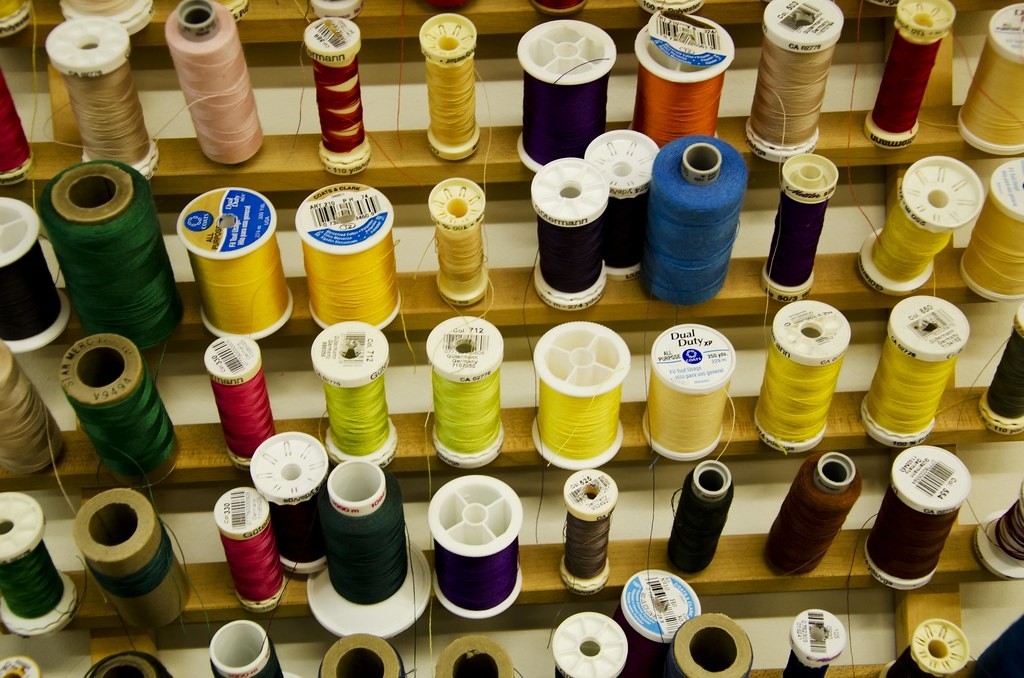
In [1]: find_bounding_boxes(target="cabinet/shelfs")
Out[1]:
[1,0,1022,677]
